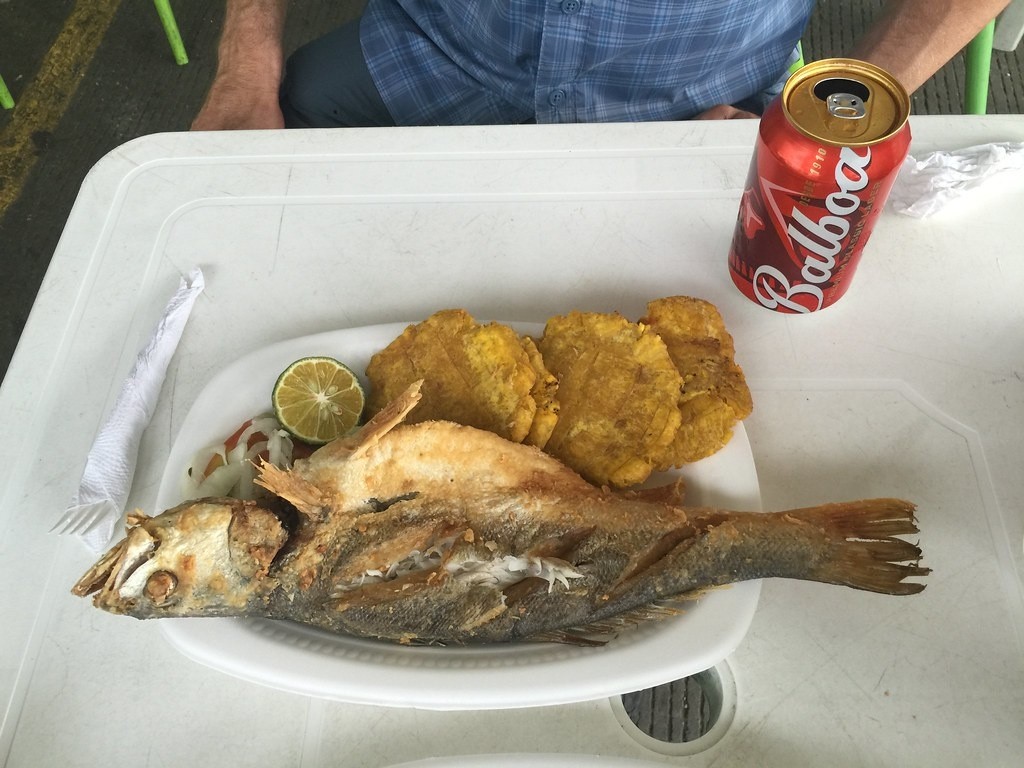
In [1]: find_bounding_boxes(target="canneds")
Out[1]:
[727,56,912,314]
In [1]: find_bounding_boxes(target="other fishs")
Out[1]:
[71,379,933,647]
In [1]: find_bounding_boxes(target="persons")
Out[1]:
[191,0,1014,132]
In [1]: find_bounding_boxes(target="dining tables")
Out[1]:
[0,114,1024,768]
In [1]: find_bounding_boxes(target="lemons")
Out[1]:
[272,356,365,446]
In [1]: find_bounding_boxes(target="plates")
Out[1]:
[147,320,764,712]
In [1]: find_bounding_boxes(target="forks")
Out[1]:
[46,488,130,540]
